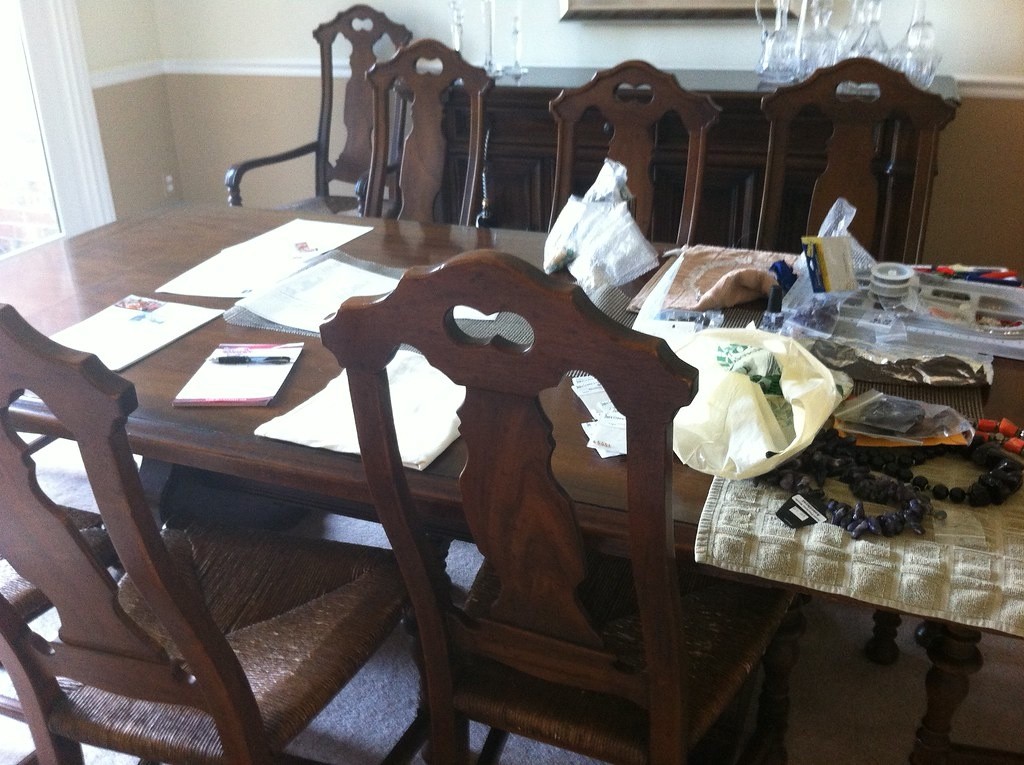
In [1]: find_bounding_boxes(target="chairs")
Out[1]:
[355,36,496,233]
[752,56,960,268]
[548,57,722,248]
[225,2,415,220]
[320,249,815,765]
[0,301,436,765]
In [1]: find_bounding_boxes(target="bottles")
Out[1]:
[475,198,501,229]
[754,0,941,91]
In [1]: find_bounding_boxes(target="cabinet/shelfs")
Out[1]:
[390,66,964,260]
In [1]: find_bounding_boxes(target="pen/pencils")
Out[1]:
[205,355,291,364]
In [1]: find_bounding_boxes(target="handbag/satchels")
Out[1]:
[668,328,854,480]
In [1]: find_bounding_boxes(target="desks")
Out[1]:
[0,202,1024,765]
